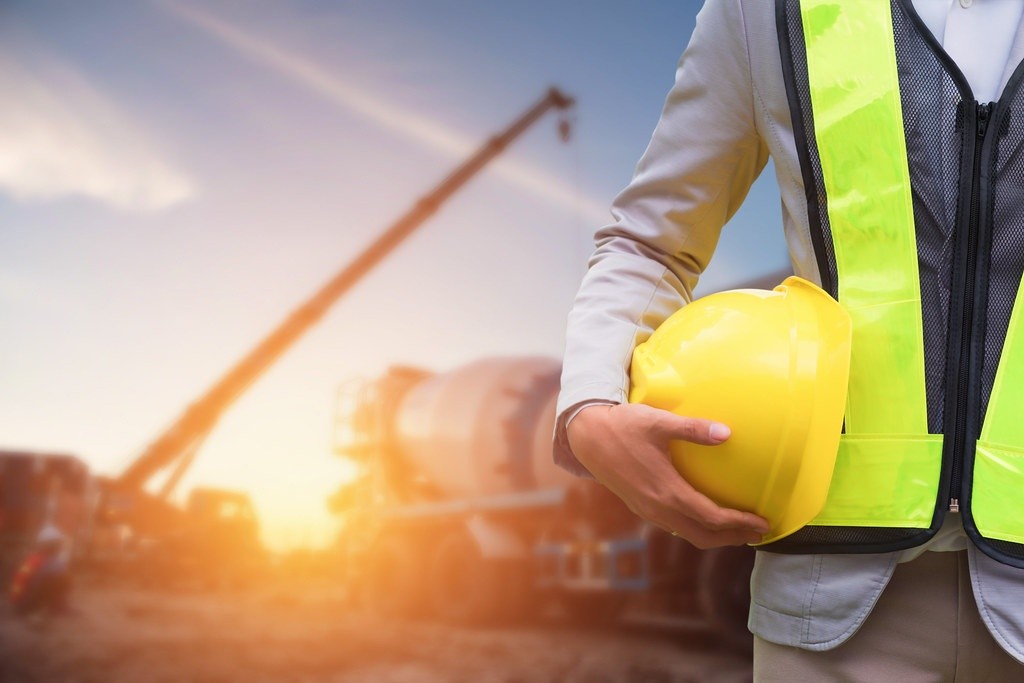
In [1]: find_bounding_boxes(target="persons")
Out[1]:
[550,0,1024,683]
[9,539,75,615]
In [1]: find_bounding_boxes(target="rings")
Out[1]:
[672,530,680,537]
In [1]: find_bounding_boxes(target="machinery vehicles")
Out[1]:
[78,84,575,588]
[320,353,756,659]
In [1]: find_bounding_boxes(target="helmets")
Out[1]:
[628,276,851,546]
